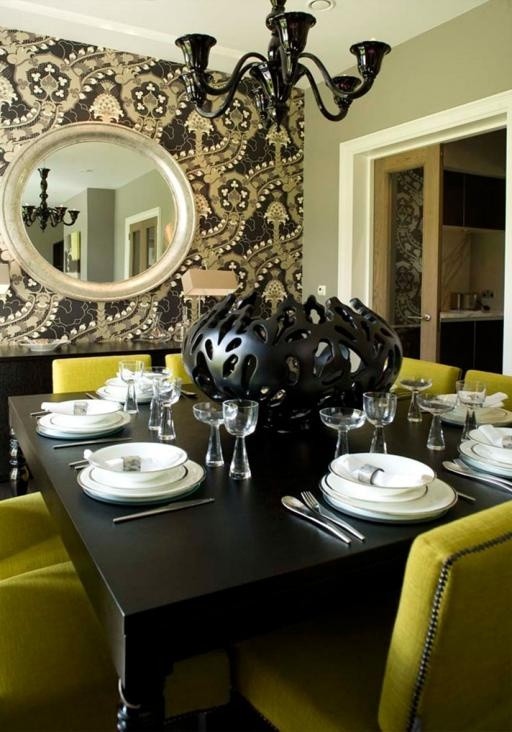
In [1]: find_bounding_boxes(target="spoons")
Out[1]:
[442,461,512,494]
[180,389,197,397]
[280,495,353,545]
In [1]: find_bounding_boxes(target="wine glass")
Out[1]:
[362,391,397,453]
[118,359,182,442]
[192,401,236,468]
[319,406,367,460]
[397,375,487,451]
[222,399,259,481]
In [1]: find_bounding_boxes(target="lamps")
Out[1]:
[20,159,81,232]
[173,0,391,135]
[181,267,239,320]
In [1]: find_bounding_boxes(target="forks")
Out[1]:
[301,491,365,541]
[452,458,512,487]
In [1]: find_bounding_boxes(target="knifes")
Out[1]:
[112,497,215,524]
[52,436,132,449]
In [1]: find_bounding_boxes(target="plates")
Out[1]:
[318,473,458,525]
[76,460,207,506]
[433,405,512,428]
[95,378,151,405]
[35,411,132,440]
[457,440,512,478]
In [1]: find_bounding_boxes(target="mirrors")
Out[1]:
[1,119,198,302]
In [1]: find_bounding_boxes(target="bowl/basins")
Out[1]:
[328,453,436,500]
[50,399,123,425]
[89,444,187,483]
[467,426,512,455]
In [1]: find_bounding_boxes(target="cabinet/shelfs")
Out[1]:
[440,322,502,372]
[442,170,504,231]
